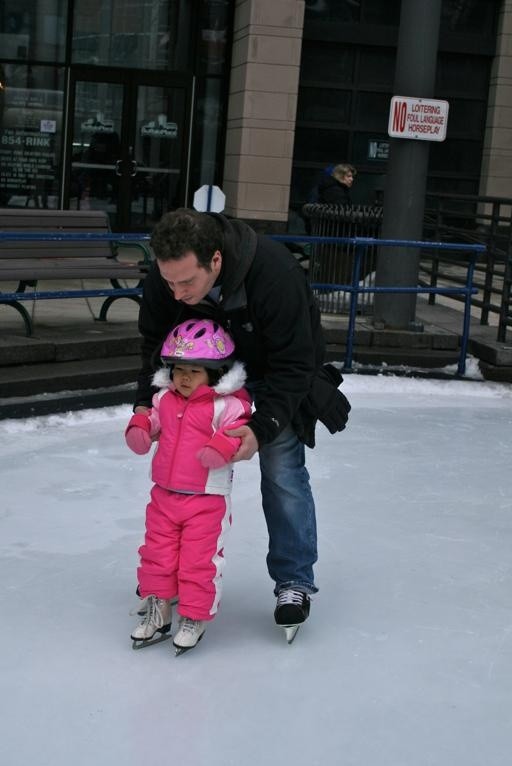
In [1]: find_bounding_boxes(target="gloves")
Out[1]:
[125,428,152,455]
[195,446,225,471]
[293,358,351,448]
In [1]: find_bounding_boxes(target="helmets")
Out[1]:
[158,316,235,375]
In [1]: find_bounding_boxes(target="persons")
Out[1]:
[316,162,358,208]
[124,318,254,650]
[132,207,351,627]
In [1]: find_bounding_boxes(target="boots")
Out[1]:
[129,591,180,642]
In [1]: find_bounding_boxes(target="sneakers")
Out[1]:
[172,616,207,649]
[271,585,313,626]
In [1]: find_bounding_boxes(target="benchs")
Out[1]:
[1,208,152,339]
